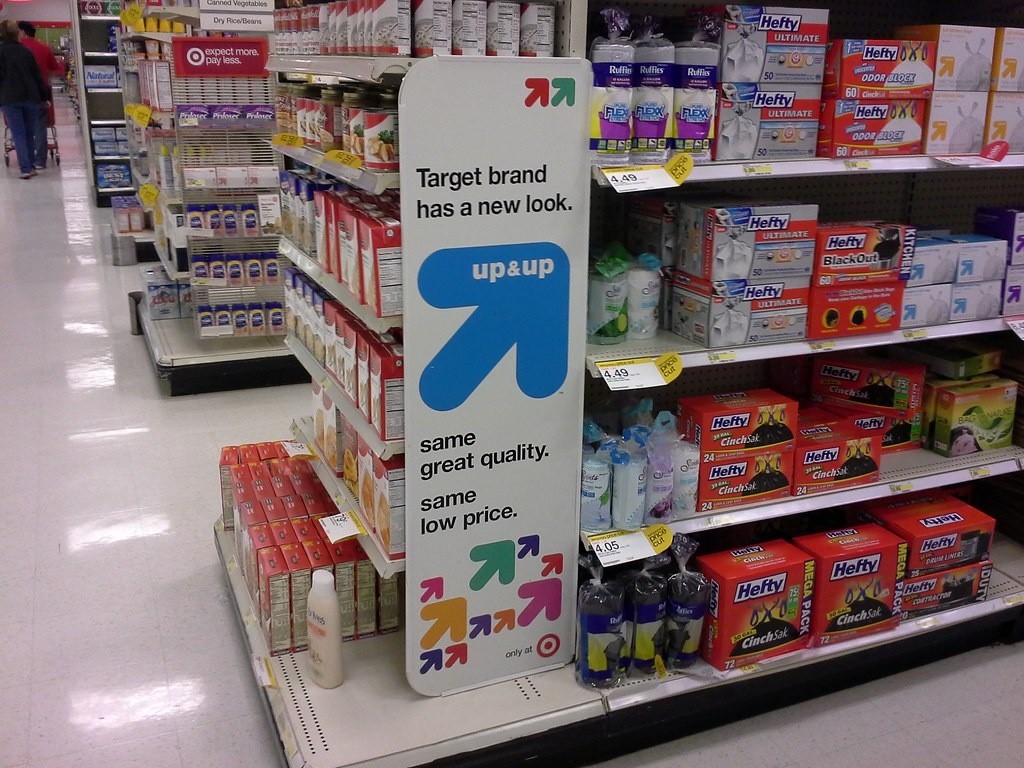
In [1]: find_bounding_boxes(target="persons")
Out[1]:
[952,427,976,455]
[0,19,70,179]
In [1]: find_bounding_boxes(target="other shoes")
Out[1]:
[18,171,39,178]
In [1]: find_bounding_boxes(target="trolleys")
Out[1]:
[3,82,61,167]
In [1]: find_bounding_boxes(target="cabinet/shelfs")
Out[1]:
[213,0,607,768]
[120,5,294,383]
[579,0,1024,740]
[72,0,137,207]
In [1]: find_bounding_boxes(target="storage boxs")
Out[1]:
[931,378,1019,458]
[711,4,1024,340]
[78,0,283,319]
[886,337,1004,380]
[696,452,794,512]
[920,374,999,450]
[809,352,927,419]
[792,523,908,648]
[658,266,674,330]
[675,196,819,282]
[900,560,994,621]
[219,440,399,658]
[676,388,799,464]
[670,270,812,348]
[848,488,997,579]
[767,349,868,396]
[791,422,882,496]
[696,538,817,673]
[822,404,925,455]
[625,191,753,279]
